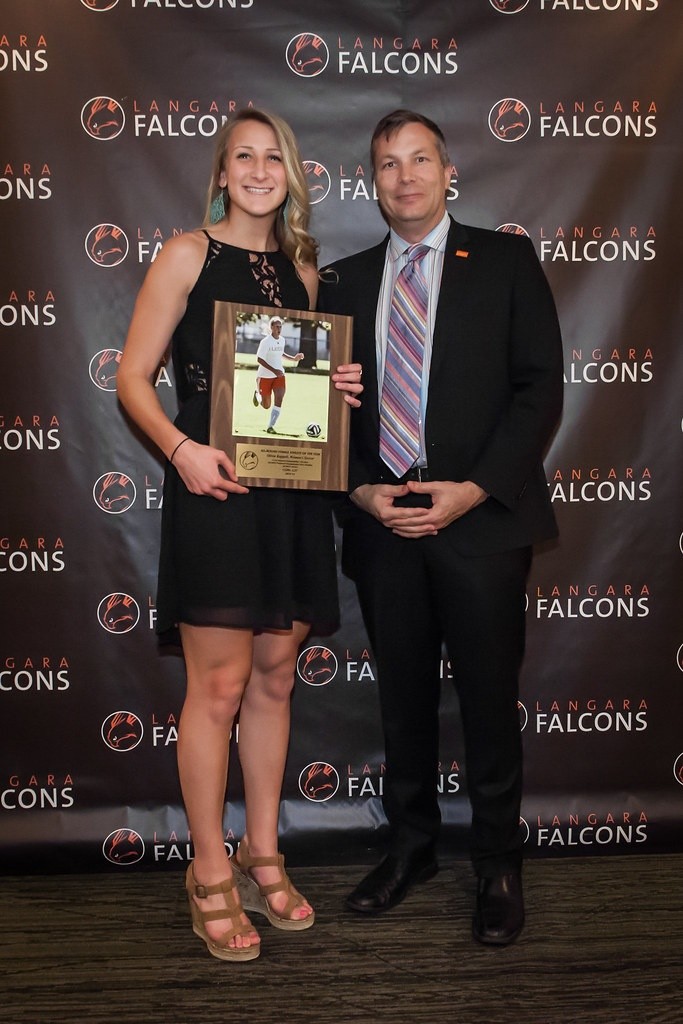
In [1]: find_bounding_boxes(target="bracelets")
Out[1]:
[170,437,191,462]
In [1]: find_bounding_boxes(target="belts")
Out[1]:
[379,466,430,482]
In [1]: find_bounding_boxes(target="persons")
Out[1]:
[311,111,565,944]
[117,109,363,963]
[253,318,306,435]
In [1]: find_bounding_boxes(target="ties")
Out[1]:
[378,243,431,480]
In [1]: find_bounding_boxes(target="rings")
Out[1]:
[359,369,363,376]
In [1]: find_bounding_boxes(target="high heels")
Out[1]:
[228,835,315,930]
[185,860,260,962]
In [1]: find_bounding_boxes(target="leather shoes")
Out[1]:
[346,851,439,912]
[471,864,525,944]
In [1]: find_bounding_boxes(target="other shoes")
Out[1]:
[267,428,276,434]
[253,390,259,407]
